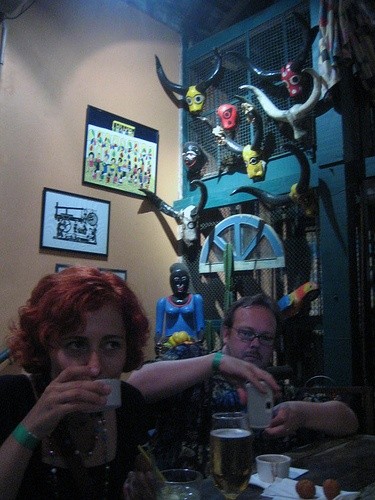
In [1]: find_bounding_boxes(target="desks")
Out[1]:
[202,434,375,500]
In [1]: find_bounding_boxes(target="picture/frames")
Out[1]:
[54,263,127,282]
[82,105,159,196]
[40,188,111,256]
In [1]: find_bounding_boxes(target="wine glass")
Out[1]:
[210,412,255,500]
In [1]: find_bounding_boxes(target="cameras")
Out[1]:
[245,381,274,429]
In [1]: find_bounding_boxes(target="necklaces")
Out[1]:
[40,392,111,500]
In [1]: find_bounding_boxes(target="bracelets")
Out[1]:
[210,350,224,376]
[11,421,42,450]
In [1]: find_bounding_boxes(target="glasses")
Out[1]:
[230,326,277,346]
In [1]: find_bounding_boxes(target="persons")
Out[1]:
[124,294,359,481]
[0,261,167,500]
[150,262,207,437]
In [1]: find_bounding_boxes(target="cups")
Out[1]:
[246,381,273,429]
[152,469,203,500]
[255,454,292,484]
[78,377,121,413]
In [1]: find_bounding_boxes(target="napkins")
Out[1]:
[250,467,360,500]
